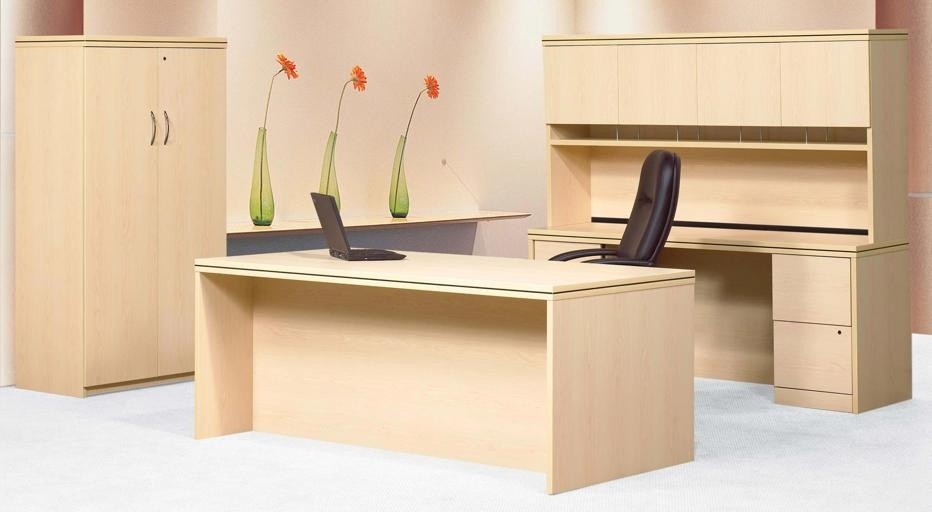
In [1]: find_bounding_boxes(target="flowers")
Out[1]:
[244,56,440,227]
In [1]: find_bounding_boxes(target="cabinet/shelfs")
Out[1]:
[523,27,913,415]
[14,34,230,398]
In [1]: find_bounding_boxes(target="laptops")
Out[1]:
[310,192,407,261]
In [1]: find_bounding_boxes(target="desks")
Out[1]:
[188,245,694,500]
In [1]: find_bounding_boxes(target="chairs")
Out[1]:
[546,151,681,268]
